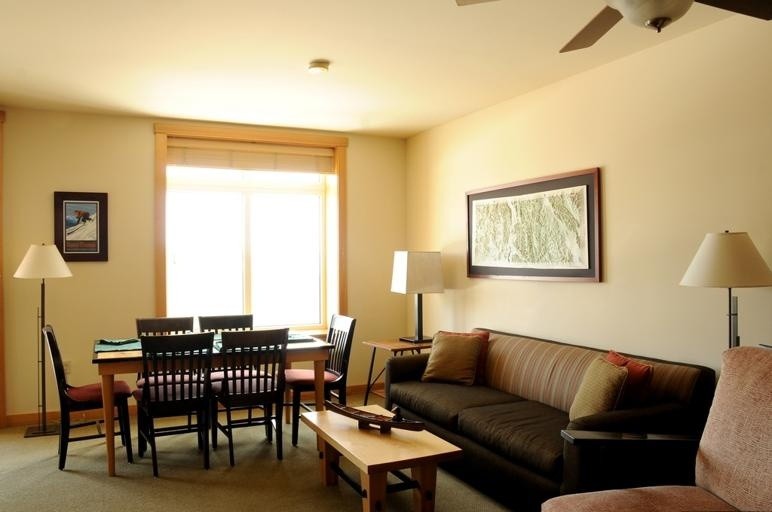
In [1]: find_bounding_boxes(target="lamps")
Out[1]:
[13,242,73,438]
[391,250,444,344]
[678,230,772,348]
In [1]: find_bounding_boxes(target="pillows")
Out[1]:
[569,355,628,421]
[422,334,482,385]
[606,350,653,393]
[438,331,489,386]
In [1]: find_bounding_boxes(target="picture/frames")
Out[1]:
[54,190,108,262]
[465,168,600,282]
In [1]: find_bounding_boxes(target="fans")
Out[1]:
[456,0,772,53]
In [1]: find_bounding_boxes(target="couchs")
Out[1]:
[539,349,772,512]
[383,328,715,512]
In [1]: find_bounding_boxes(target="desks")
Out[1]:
[92,336,336,477]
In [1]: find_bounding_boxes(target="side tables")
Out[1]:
[363,337,432,405]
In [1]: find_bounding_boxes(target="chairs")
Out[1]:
[212,328,289,466]
[137,317,206,387]
[43,324,134,470]
[199,314,272,381]
[275,313,357,446]
[132,332,215,477]
